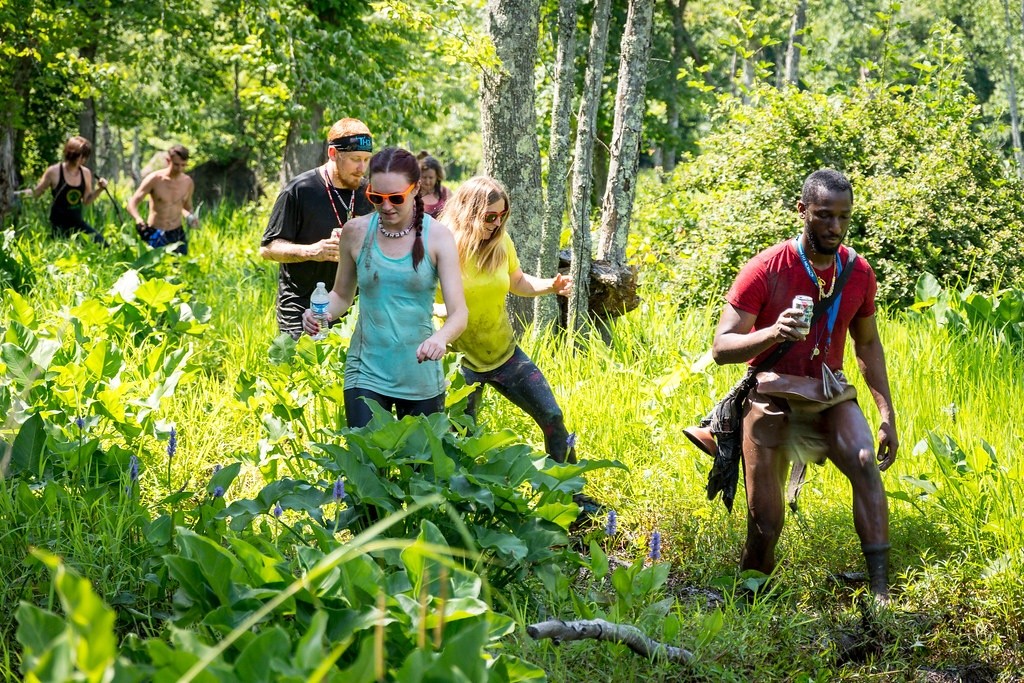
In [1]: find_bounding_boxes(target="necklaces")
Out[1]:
[378,216,415,239]
[808,254,836,300]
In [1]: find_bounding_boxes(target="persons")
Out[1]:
[127,145,200,259]
[260,119,372,341]
[303,147,469,526]
[415,151,454,220]
[437,176,606,515]
[14,136,111,249]
[713,169,899,599]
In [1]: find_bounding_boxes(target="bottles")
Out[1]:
[310,281,330,340]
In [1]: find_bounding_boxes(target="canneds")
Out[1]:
[330,228,343,240]
[789,295,813,336]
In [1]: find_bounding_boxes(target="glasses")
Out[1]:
[171,160,189,167]
[366,183,415,206]
[485,211,506,223]
